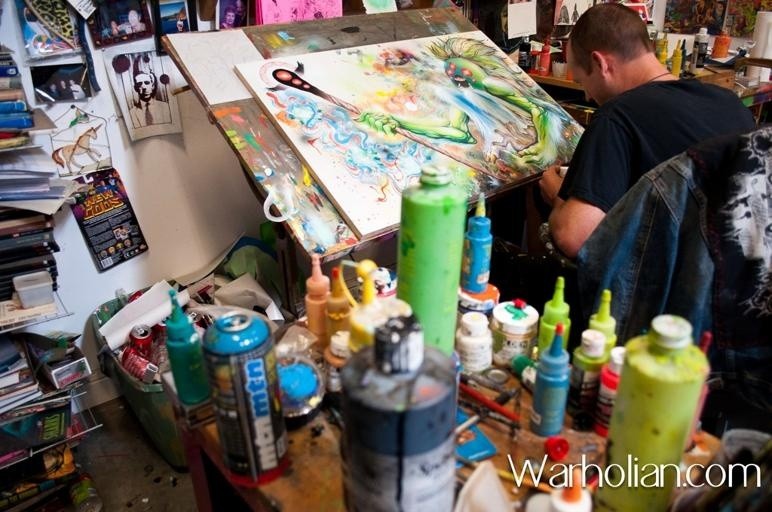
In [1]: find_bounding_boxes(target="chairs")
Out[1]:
[735,57,772,124]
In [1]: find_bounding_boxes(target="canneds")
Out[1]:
[152,321,170,377]
[129,323,152,359]
[121,345,150,379]
[128,288,142,301]
[187,309,207,327]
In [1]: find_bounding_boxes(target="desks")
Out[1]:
[527,67,772,127]
[162,363,721,512]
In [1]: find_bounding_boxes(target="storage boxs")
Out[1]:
[92,273,297,472]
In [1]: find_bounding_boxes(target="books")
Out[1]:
[0,335,43,415]
[0,44,84,301]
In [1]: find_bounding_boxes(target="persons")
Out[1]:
[111,21,120,36]
[69,79,86,100]
[58,80,73,99]
[49,84,59,100]
[177,18,183,32]
[220,7,235,29]
[234,0,246,26]
[128,10,145,33]
[129,72,171,128]
[538,2,757,256]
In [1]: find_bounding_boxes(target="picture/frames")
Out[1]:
[152,0,190,56]
[88,0,151,48]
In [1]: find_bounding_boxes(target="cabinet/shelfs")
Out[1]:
[0,291,104,511]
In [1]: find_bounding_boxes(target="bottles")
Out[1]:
[163,162,711,512]
[691,27,710,69]
[654,27,686,75]
[67,463,104,512]
[710,27,731,58]
[518,35,551,76]
